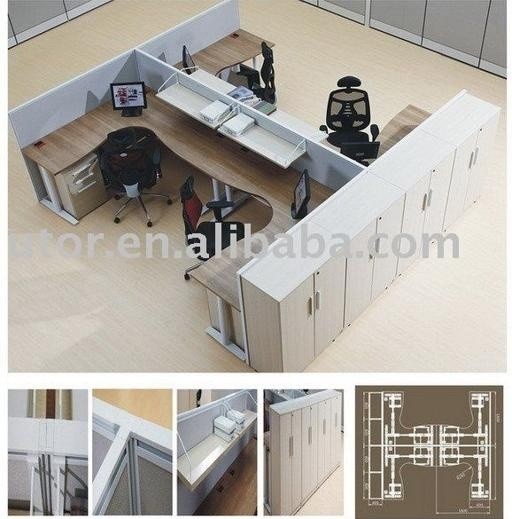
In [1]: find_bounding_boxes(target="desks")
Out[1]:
[172,29,432,165]
[19,81,336,364]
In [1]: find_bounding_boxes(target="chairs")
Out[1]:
[179,174,254,281]
[94,123,173,230]
[318,75,379,147]
[248,42,277,106]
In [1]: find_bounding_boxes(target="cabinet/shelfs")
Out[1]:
[268,390,342,515]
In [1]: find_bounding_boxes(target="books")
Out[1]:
[253,100,277,116]
[227,85,253,102]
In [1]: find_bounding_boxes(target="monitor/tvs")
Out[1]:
[182,45,195,74]
[340,142,380,167]
[110,81,147,117]
[290,169,312,220]
